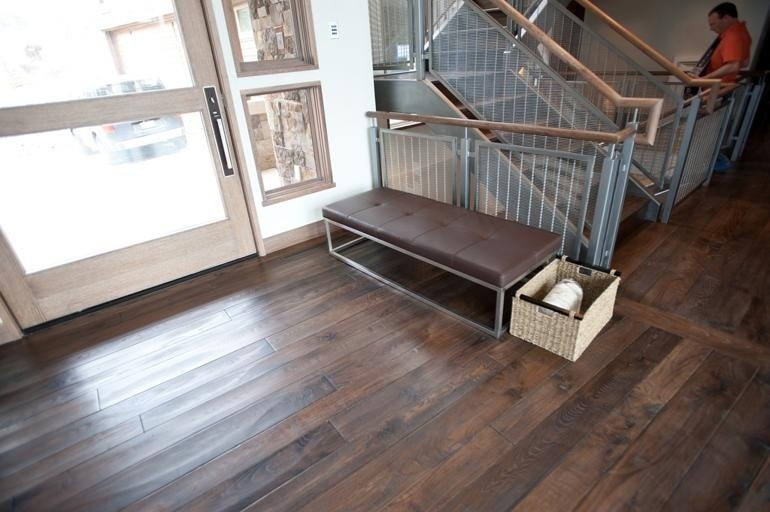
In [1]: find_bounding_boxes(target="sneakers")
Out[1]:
[713,153,734,173]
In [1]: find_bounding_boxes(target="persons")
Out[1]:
[682,2,752,173]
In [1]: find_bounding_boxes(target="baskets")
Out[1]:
[509,255,622,362]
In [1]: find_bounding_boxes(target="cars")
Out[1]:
[66,70,186,162]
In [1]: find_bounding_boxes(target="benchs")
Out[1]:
[320,185,564,342]
